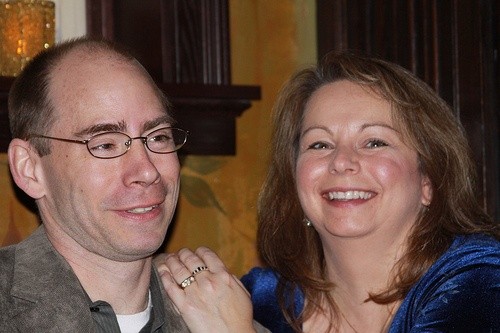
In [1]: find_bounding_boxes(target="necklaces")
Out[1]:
[339,300,399,333]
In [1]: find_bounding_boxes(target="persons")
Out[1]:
[160,49,500,333]
[0,36,273,333]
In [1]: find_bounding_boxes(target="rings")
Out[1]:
[179,266,209,289]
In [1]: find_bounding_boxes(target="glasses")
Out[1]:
[22,126,188,158]
